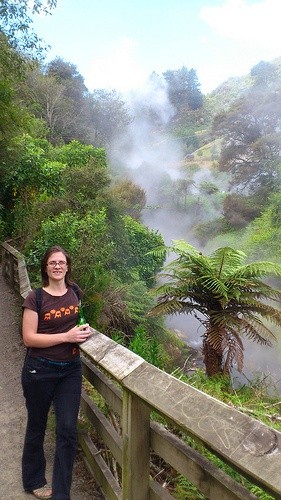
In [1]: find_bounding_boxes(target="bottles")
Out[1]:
[77,305,85,331]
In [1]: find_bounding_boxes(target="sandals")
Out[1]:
[33,484,52,499]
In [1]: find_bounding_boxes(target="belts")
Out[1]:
[35,357,80,366]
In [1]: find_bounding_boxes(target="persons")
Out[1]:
[20,245,91,500]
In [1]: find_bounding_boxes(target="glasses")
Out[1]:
[44,262,69,266]
